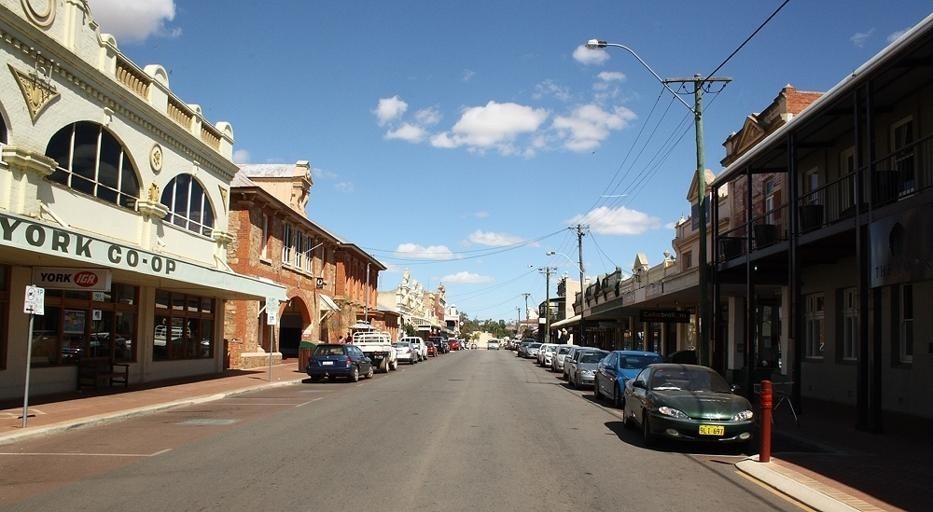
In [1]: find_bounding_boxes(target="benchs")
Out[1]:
[76,356,130,394]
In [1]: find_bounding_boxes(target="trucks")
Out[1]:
[351,330,398,373]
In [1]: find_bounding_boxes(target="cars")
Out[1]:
[424,336,460,357]
[391,342,419,365]
[303,341,373,383]
[487,338,500,350]
[514,341,759,455]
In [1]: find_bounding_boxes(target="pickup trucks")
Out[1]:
[401,337,429,362]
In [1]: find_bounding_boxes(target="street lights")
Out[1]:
[584,36,735,364]
[545,249,586,345]
